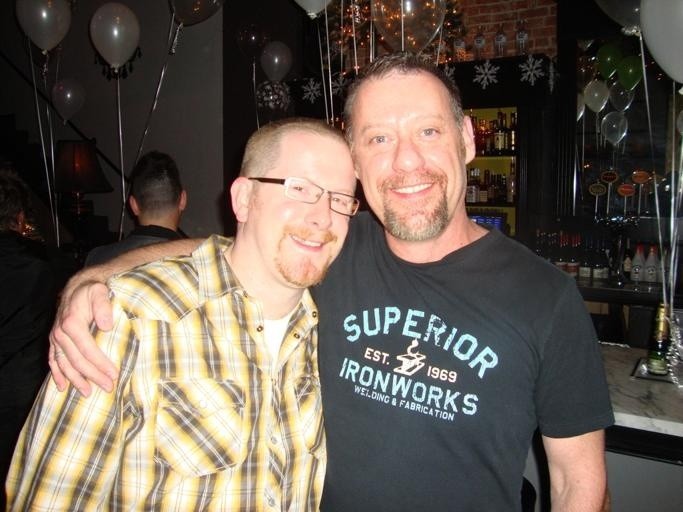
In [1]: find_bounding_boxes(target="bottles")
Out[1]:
[433,30,447,62]
[470,111,517,156]
[452,26,466,61]
[532,230,609,280]
[473,25,487,61]
[465,160,515,204]
[628,242,670,282]
[646,302,669,376]
[493,23,507,57]
[515,20,529,57]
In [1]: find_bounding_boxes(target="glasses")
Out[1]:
[246,175,360,219]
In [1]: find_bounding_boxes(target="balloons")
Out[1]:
[640,0,683,83]
[260,40,293,84]
[596,0,640,31]
[295,0,331,20]
[89,3,140,73]
[237,22,264,58]
[584,42,642,154]
[370,0,446,56]
[255,80,291,116]
[170,0,218,25]
[15,0,72,53]
[51,79,85,121]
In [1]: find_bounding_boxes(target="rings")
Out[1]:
[54,351,63,360]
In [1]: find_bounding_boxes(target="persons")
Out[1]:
[4,121,357,512]
[0,175,54,480]
[47,52,616,512]
[84,148,192,268]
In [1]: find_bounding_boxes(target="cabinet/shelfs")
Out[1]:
[288,53,574,254]
[531,164,683,356]
